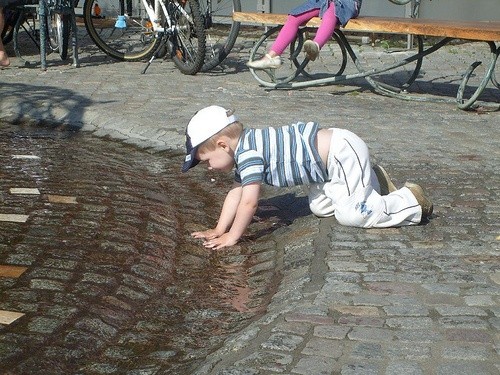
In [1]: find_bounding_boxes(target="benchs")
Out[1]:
[232,11,500,109]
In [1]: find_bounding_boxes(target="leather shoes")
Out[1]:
[247,54,281,69]
[304,40,319,61]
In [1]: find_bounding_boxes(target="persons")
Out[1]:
[0,0,10,66]
[182,105,433,251]
[245,0,364,70]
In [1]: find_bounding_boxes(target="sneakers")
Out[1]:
[405,180,433,217]
[372,164,398,196]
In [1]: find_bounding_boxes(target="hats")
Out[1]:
[181,105,239,173]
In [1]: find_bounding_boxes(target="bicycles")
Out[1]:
[2,0,29,43]
[150,0,242,73]
[45,0,71,59]
[82,0,206,77]
[13,0,61,65]
